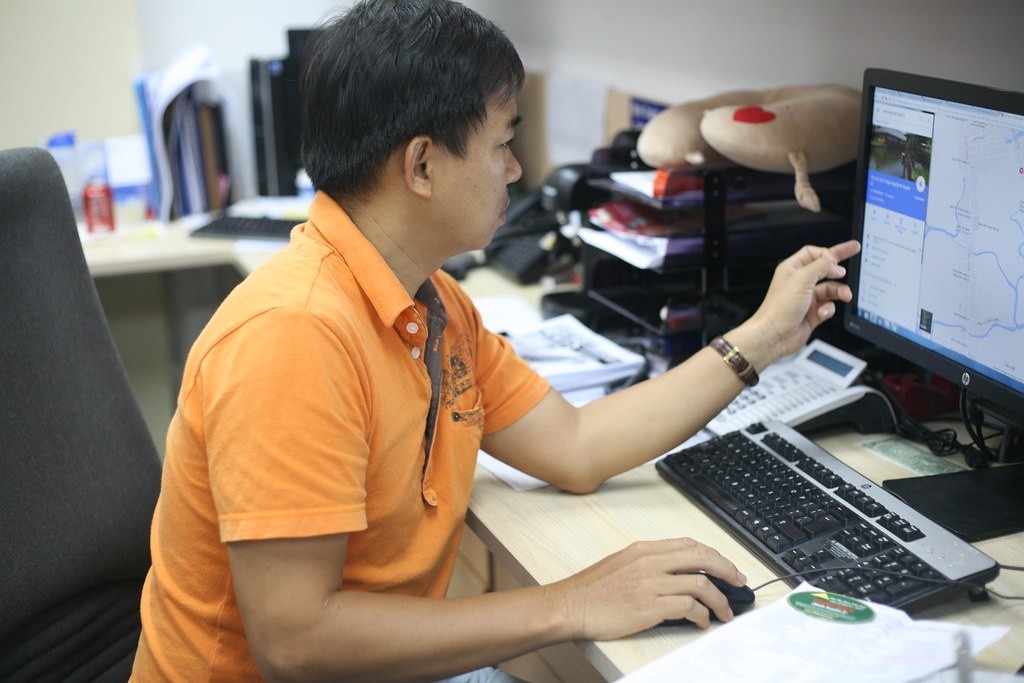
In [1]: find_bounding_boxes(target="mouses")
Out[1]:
[657,572,755,626]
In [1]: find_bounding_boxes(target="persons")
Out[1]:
[121,1,860,683]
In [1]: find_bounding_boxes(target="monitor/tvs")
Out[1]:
[838,66,1024,545]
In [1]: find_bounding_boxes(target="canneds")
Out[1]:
[84,185,114,232]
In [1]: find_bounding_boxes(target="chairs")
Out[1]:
[0,148,163,683]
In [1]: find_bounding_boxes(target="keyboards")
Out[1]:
[654,419,1000,616]
[190,216,308,240]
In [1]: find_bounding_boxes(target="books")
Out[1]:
[197,97,231,211]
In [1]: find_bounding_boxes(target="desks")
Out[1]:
[77,193,316,275]
[237,253,1023,683]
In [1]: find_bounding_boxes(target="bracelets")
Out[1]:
[709,336,762,387]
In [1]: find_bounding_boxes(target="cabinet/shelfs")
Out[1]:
[577,121,868,375]
